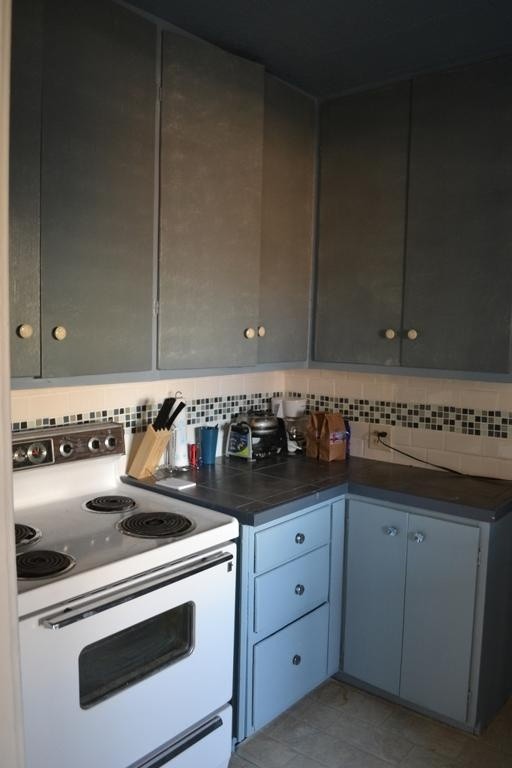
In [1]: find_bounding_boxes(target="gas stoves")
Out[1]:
[10,421,241,578]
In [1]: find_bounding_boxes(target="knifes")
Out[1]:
[153,396,184,432]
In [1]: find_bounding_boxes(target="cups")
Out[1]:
[198,427,217,463]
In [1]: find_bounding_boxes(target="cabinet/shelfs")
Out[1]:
[340,497,482,726]
[157,17,318,376]
[11,2,155,380]
[367,421,392,451]
[314,48,510,376]
[244,492,345,732]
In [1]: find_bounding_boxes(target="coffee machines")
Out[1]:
[269,395,308,457]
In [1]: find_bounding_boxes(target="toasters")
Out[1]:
[226,409,287,462]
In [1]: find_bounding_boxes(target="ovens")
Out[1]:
[17,541,238,768]
